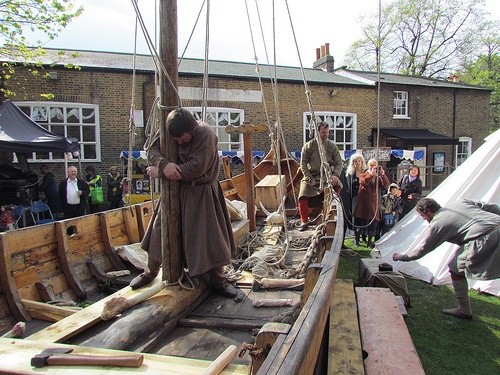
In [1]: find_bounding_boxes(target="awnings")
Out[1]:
[373,127,459,146]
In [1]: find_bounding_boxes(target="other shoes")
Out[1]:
[213,279,238,298]
[129,272,155,288]
[298,225,309,231]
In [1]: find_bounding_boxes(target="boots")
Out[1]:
[368,236,375,248]
[362,234,367,242]
[354,234,360,247]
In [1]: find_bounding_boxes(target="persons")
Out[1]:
[296,122,422,249]
[37,161,146,220]
[130,107,238,298]
[392,198,500,319]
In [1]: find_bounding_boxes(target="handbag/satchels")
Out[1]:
[91,183,104,206]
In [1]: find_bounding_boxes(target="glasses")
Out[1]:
[113,170,116,171]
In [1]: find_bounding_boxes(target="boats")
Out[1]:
[1,0,344,375]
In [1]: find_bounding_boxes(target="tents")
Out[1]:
[0,100,78,179]
[375,129,500,297]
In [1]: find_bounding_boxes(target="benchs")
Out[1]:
[357,257,409,308]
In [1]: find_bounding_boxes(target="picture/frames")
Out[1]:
[433,152,446,173]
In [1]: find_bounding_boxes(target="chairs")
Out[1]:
[31,202,56,225]
[0,205,24,232]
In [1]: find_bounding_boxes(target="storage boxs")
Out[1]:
[254,175,288,210]
[230,219,251,247]
